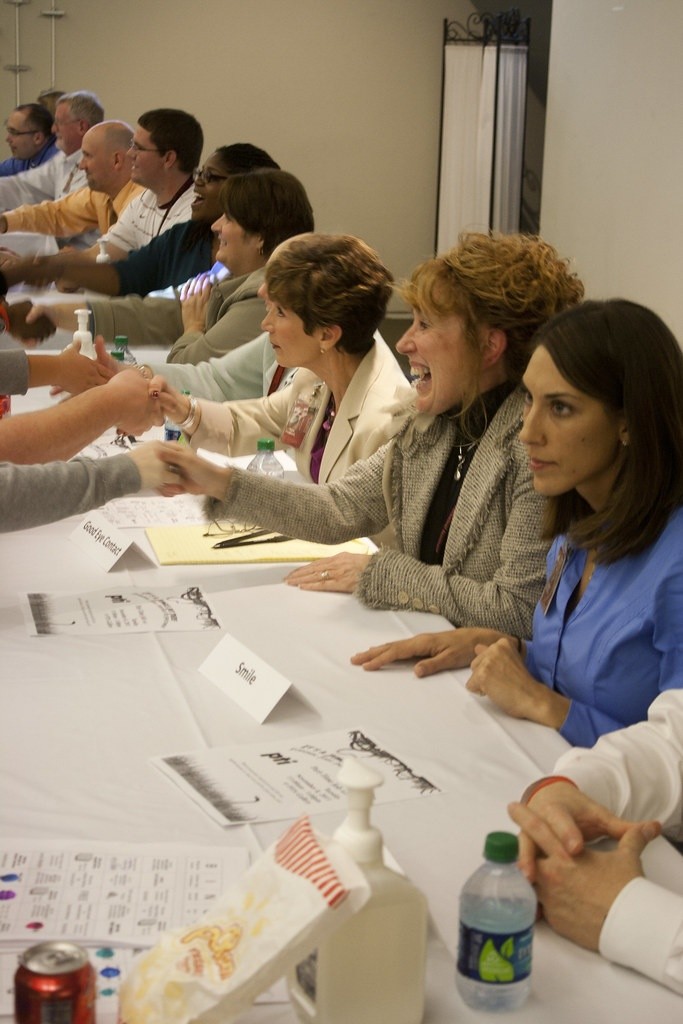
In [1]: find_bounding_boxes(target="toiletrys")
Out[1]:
[67,307,100,363]
[287,753,425,1020]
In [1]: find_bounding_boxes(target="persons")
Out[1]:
[0,369,164,464]
[0,340,114,395]
[22,168,315,366]
[50,232,411,547]
[158,234,583,634]
[353,298,683,748]
[508,688,683,995]
[0,90,277,347]
[0,439,184,534]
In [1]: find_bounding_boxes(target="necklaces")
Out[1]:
[454,441,475,481]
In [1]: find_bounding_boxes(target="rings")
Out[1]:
[169,464,177,473]
[321,569,328,580]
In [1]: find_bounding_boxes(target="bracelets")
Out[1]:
[520,776,579,806]
[173,398,196,429]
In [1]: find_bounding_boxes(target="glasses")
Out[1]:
[193,166,229,184]
[203,519,262,536]
[129,139,169,154]
[3,123,38,137]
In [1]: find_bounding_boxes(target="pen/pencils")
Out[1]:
[212,527,274,548]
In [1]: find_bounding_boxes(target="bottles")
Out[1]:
[247,438,284,480]
[110,334,140,368]
[453,832,535,1013]
[164,389,191,442]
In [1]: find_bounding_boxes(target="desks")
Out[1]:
[0,231,683,1024]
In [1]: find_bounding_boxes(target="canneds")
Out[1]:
[13,941,97,1024]
